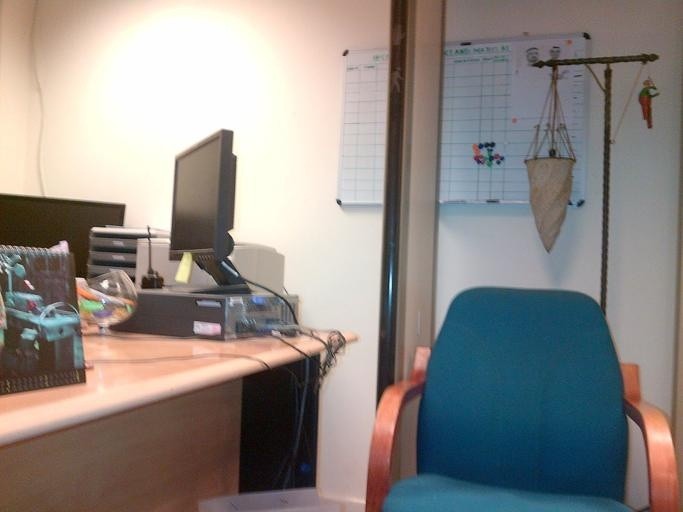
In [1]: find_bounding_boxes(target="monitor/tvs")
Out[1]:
[168,129,254,295]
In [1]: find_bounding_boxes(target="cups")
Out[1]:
[84,270,136,338]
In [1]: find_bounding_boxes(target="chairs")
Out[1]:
[366,287,680,512]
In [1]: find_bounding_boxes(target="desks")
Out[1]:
[0,324,359,512]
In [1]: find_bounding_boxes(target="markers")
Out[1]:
[487,199,499,203]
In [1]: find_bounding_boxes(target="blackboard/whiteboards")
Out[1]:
[336,32,593,208]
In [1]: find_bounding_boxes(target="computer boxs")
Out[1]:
[109,283,300,342]
